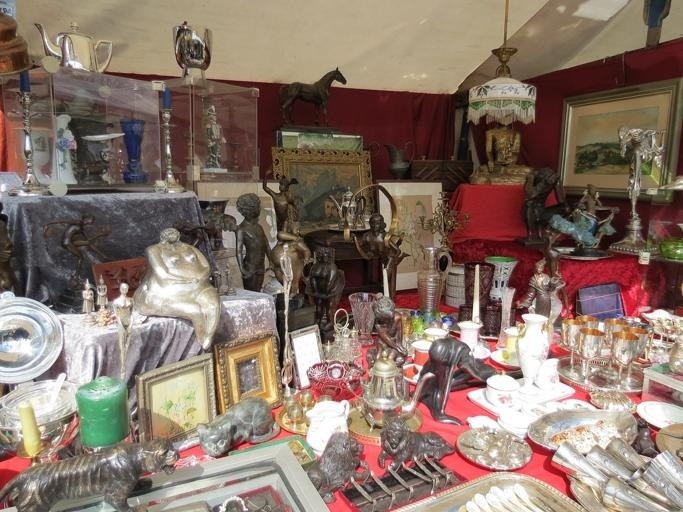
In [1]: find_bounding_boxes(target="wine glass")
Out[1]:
[273,389,334,434]
[550,303,683,393]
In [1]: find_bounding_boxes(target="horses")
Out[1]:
[278,67,348,126]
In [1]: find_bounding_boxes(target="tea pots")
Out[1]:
[303,399,350,458]
[517,310,552,383]
[327,184,366,230]
[412,246,453,314]
[31,19,114,76]
[172,21,212,91]
[343,350,436,432]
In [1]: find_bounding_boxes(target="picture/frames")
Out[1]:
[134,353,216,450]
[215,331,283,414]
[376,179,444,295]
[556,78,683,202]
[272,147,373,236]
[289,325,324,391]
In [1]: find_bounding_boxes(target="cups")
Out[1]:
[485,373,520,410]
[647,219,672,258]
[307,361,366,401]
[532,357,561,390]
[403,258,520,385]
[347,291,379,346]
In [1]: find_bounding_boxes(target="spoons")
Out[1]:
[457,482,545,512]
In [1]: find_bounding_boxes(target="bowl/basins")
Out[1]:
[660,239,683,261]
[0,380,78,457]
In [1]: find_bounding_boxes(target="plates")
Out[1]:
[569,451,654,510]
[466,378,577,416]
[0,290,63,385]
[457,398,682,475]
[346,398,425,446]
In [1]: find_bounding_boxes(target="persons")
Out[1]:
[521,166,621,249]
[205,104,227,168]
[516,258,562,317]
[83,170,408,378]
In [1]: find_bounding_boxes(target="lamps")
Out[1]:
[468,0,537,125]
